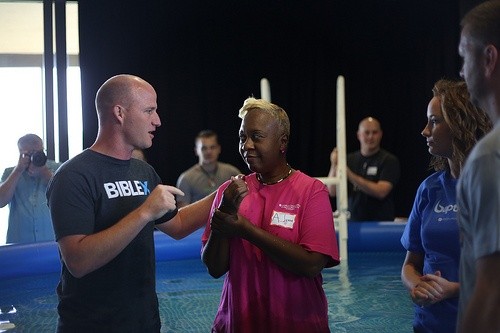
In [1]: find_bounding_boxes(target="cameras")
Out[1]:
[23,150,47,171]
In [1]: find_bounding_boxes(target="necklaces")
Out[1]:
[255,162,292,184]
[200,163,220,187]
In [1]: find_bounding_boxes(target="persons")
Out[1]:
[400,78,492,333]
[0,133,65,243]
[326,116,403,221]
[455,0,499,332]
[46,74,247,333]
[199,97,341,332]
